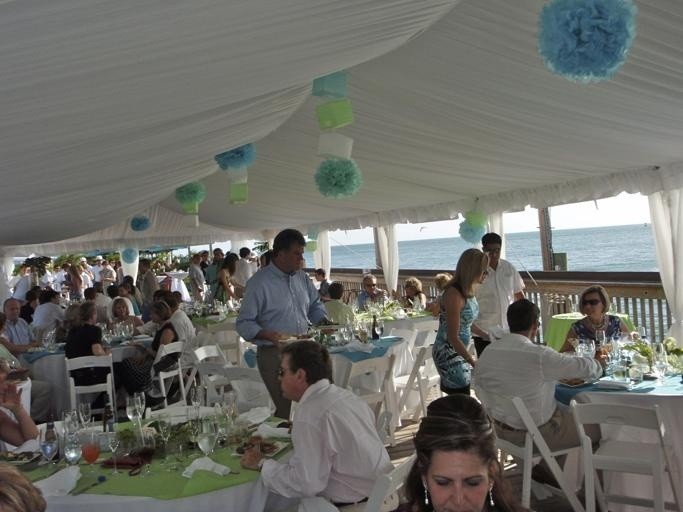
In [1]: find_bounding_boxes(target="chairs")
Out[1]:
[0,293,681,511]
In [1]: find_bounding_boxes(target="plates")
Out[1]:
[278,336,316,344]
[557,380,588,388]
[8,453,40,465]
[307,325,344,330]
[231,442,289,457]
[249,414,271,427]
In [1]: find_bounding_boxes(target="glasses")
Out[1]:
[482,247,500,254]
[582,298,602,306]
[406,285,416,288]
[277,366,289,377]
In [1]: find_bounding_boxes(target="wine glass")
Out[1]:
[42,323,135,353]
[38,386,237,474]
[375,321,384,344]
[195,307,203,320]
[577,327,667,387]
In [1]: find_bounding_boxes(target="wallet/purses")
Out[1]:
[104,455,150,476]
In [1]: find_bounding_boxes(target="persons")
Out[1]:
[559,286,632,354]
[314,269,450,325]
[240,342,396,512]
[0,460,47,512]
[431,248,494,395]
[0,247,272,447]
[389,393,528,511]
[236,229,334,419]
[470,298,601,488]
[471,234,527,356]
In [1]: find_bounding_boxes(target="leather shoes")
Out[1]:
[532,464,564,494]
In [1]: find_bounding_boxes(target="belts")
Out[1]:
[493,407,558,432]
[333,496,368,508]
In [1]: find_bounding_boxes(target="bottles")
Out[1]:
[371,315,380,339]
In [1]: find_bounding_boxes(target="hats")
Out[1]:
[81,257,87,262]
[92,255,106,261]
